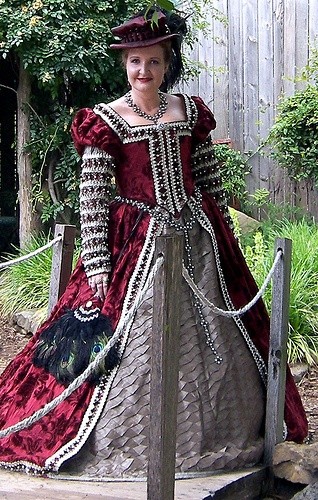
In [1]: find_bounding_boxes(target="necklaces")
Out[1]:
[123,90,169,125]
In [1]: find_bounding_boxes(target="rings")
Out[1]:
[90,277,109,284]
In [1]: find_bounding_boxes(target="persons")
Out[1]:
[0,6,311,482]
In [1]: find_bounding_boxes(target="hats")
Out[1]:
[109,5,188,48]
